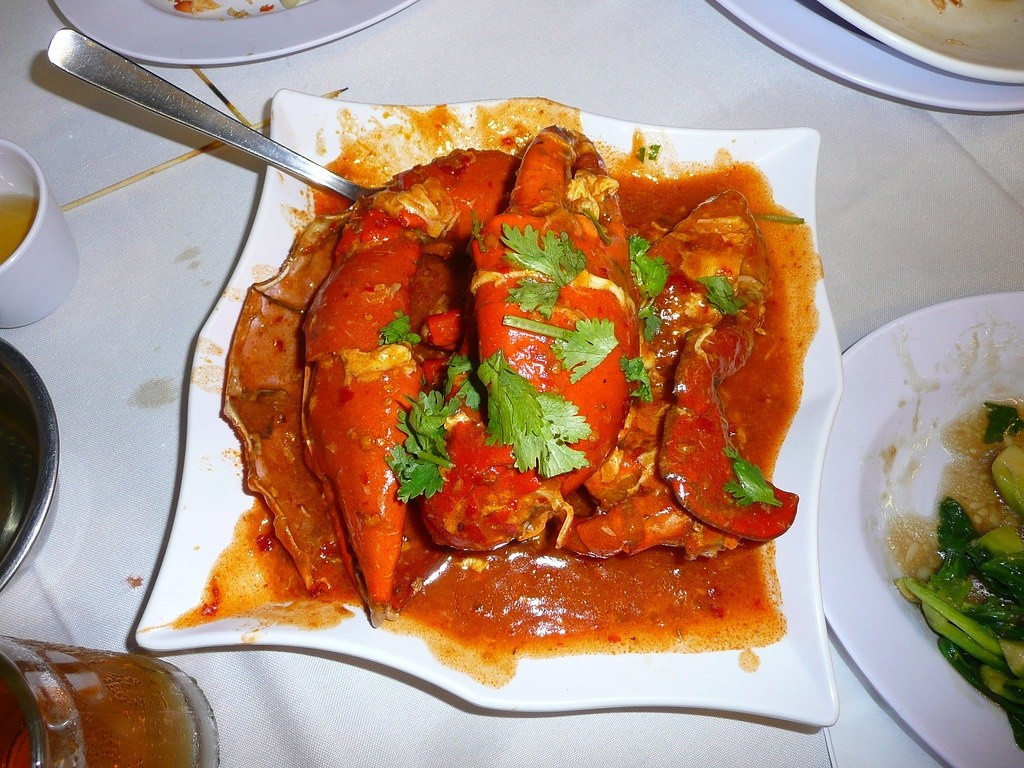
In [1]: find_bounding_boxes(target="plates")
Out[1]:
[132,87,845,728]
[817,290,1024,768]
[714,0,1024,113]
[53,0,419,65]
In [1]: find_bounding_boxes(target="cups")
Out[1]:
[0,632,220,768]
[0,136,79,329]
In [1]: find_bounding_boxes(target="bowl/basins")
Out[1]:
[815,0,1024,85]
[0,337,60,591]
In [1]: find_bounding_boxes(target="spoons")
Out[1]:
[46,29,475,263]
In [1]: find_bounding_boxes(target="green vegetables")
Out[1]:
[895,399,1024,751]
[376,140,785,510]
